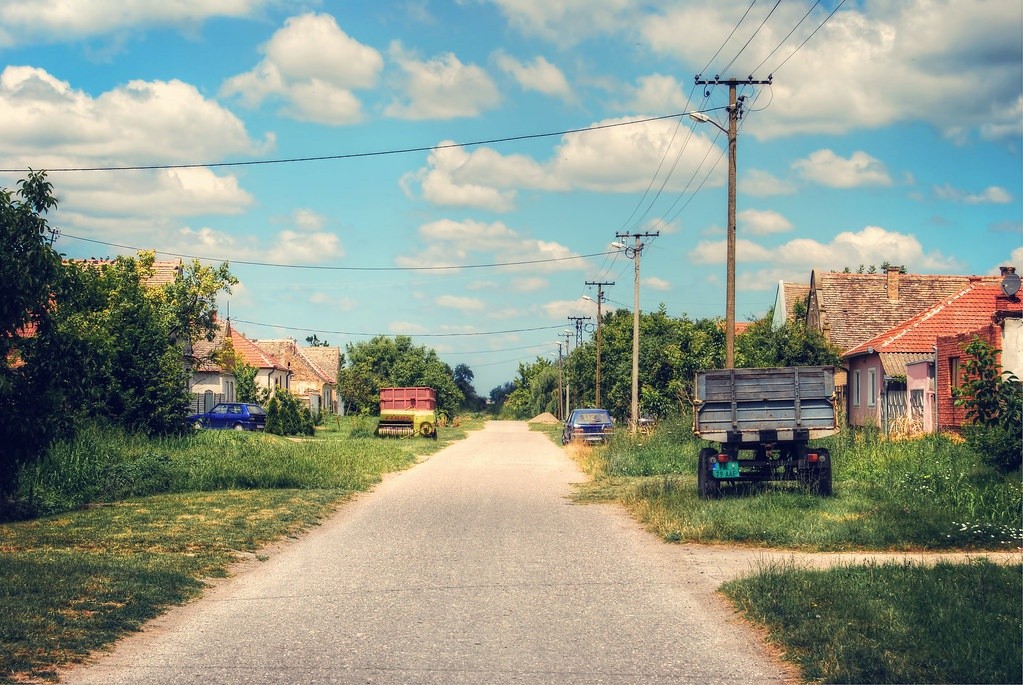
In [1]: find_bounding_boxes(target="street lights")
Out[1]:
[582,295,601,406]
[612,240,640,434]
[564,329,573,420]
[688,108,736,371]
[556,340,562,421]
[551,352,559,419]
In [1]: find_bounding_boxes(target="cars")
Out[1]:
[562,409,615,448]
[186,402,267,434]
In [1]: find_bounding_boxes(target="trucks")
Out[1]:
[691,364,842,499]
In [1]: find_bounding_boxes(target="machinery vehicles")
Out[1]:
[375,386,437,440]
